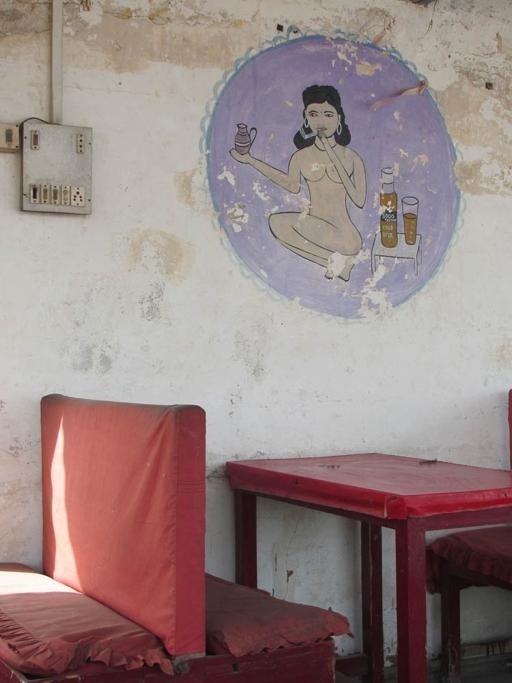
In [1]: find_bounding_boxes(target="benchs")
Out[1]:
[426,393,512,681]
[0,391,345,682]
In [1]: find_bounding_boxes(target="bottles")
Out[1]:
[378,166,399,249]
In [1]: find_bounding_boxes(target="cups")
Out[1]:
[400,195,420,246]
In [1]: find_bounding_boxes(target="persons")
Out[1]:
[228,85,366,283]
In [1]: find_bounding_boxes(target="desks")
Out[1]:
[221,455,512,682]
